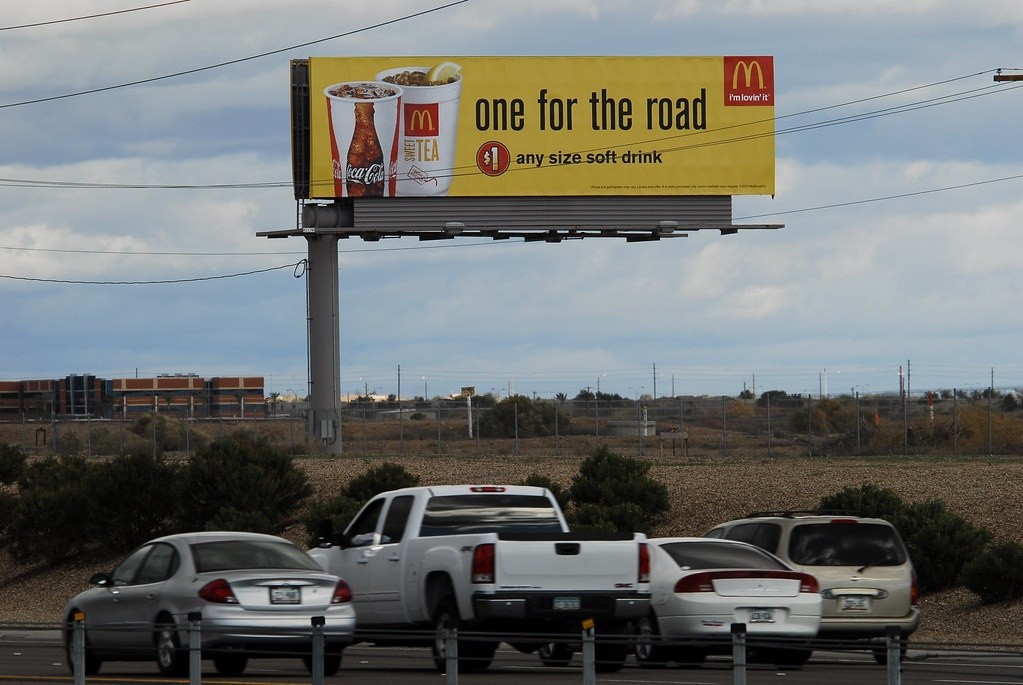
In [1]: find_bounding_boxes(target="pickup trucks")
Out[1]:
[301,483,658,675]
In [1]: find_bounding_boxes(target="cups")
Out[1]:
[375,67,462,197]
[323,81,404,198]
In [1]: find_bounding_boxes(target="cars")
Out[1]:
[628,538,822,666]
[61,531,355,675]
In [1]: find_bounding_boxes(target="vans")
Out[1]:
[698,510,919,666]
